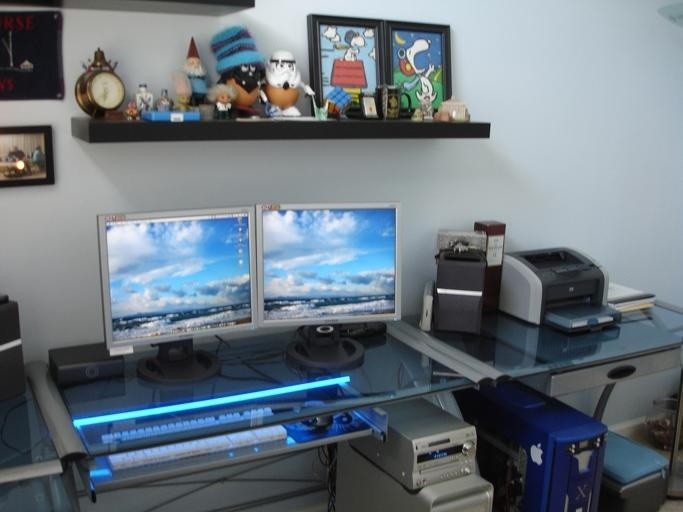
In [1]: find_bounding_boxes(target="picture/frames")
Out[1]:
[0,125,56,188]
[0,9,65,101]
[306,13,453,121]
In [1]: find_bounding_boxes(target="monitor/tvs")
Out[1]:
[96,206,256,385]
[256,202,401,371]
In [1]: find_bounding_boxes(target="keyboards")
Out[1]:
[102,408,288,470]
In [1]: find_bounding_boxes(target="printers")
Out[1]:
[502,246,622,334]
[495,312,621,364]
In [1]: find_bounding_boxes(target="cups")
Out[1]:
[378,85,412,120]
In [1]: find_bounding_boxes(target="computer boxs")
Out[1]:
[480,386,606,511]
[335,445,494,511]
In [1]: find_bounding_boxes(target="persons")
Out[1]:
[205,84,238,120]
[419,95,434,120]
[173,37,208,114]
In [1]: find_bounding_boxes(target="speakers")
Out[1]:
[0,301,27,403]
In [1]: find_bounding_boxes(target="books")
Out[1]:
[604,280,657,310]
[615,303,657,314]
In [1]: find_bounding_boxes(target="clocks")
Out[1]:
[74,47,125,118]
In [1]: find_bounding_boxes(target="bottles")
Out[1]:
[154,88,173,112]
[136,84,154,112]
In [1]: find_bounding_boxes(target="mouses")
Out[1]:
[301,399,333,427]
